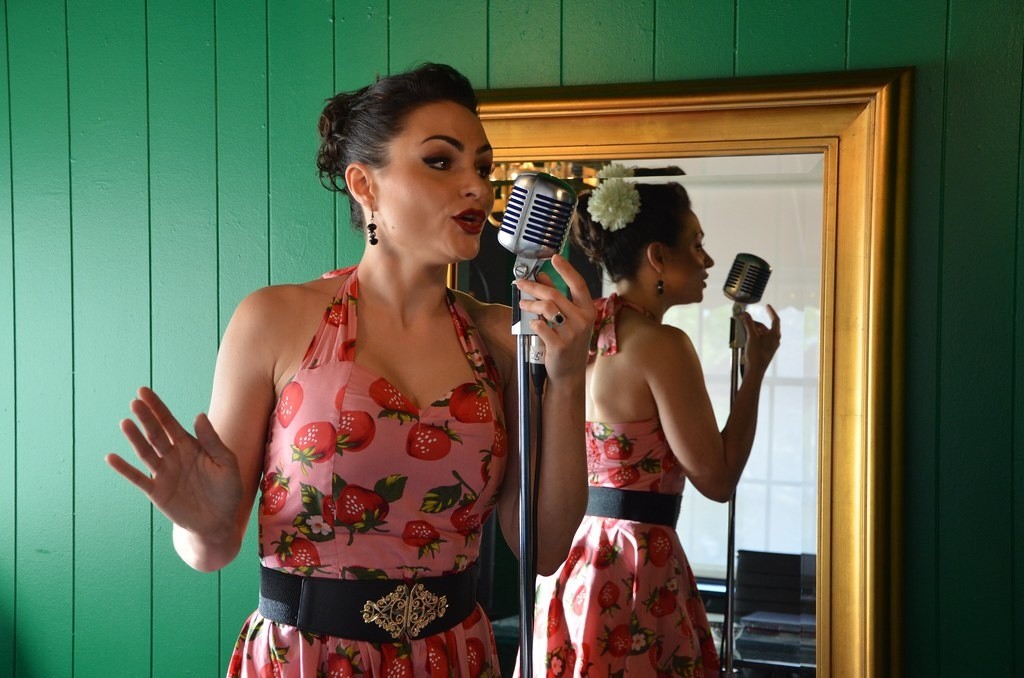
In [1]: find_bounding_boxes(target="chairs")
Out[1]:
[734,550,802,622]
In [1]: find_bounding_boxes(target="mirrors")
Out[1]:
[446,66,915,678]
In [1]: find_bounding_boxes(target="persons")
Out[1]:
[104,63,597,678]
[512,161,782,678]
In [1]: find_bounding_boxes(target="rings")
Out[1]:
[553,310,566,326]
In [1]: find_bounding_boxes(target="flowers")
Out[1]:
[587,177,642,230]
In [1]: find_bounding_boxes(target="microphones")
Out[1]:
[495,170,580,400]
[723,253,772,379]
